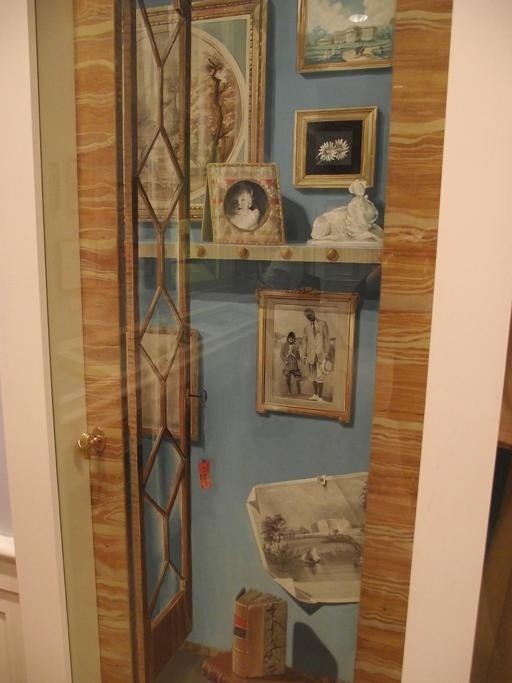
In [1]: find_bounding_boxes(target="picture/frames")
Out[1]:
[135,324,201,442]
[131,2,267,224]
[254,287,361,425]
[203,162,283,245]
[291,107,377,190]
[294,0,393,75]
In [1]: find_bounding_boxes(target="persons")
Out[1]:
[301,308,330,402]
[227,182,260,231]
[279,331,305,397]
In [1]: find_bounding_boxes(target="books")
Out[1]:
[199,587,339,683]
